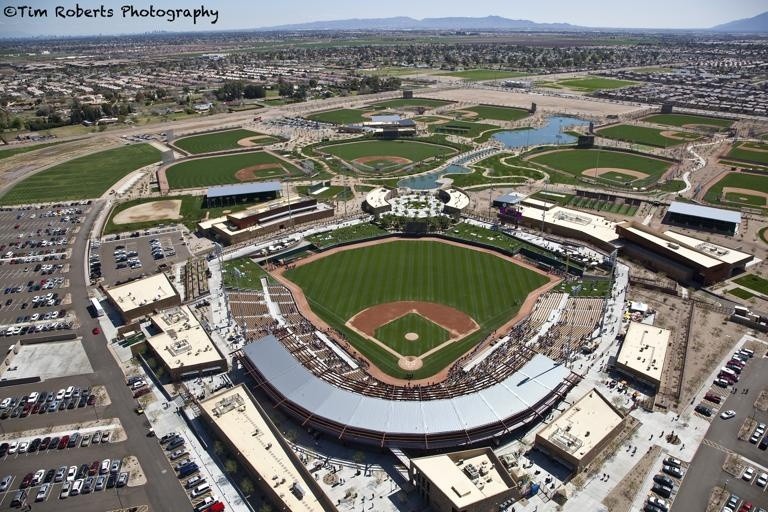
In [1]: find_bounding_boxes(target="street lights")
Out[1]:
[722,479,730,494]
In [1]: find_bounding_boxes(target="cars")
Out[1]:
[756,472,768,488]
[712,347,755,388]
[643,456,685,512]
[704,392,721,405]
[719,409,736,420]
[720,493,767,512]
[741,466,757,482]
[694,405,712,417]
[749,422,768,450]
